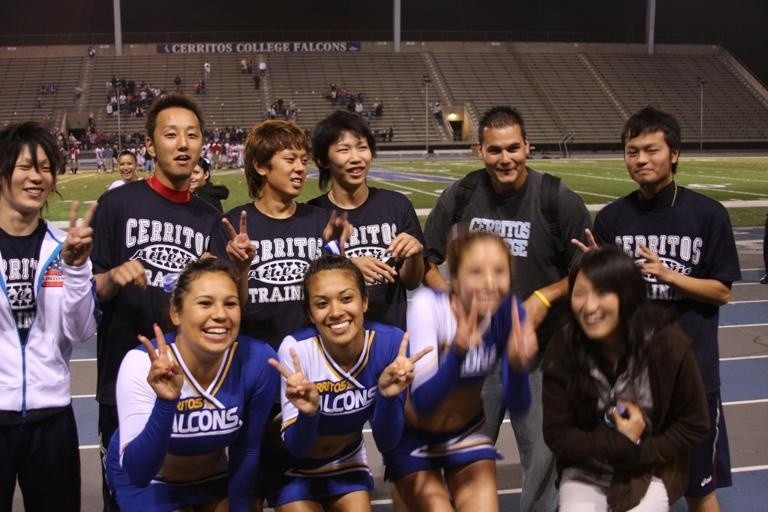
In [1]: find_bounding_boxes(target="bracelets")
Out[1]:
[535,291,550,307]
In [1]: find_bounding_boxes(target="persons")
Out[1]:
[390,231,536,511]
[572,109,743,511]
[105,257,279,512]
[422,106,593,511]
[87,97,224,511]
[37,83,55,108]
[266,98,299,121]
[540,250,709,511]
[190,126,251,210]
[54,113,155,190]
[105,75,182,119]
[329,83,393,141]
[304,112,428,511]
[268,255,433,512]
[88,46,96,56]
[1,121,98,510]
[196,61,210,94]
[757,215,768,284]
[207,120,353,512]
[241,58,267,90]
